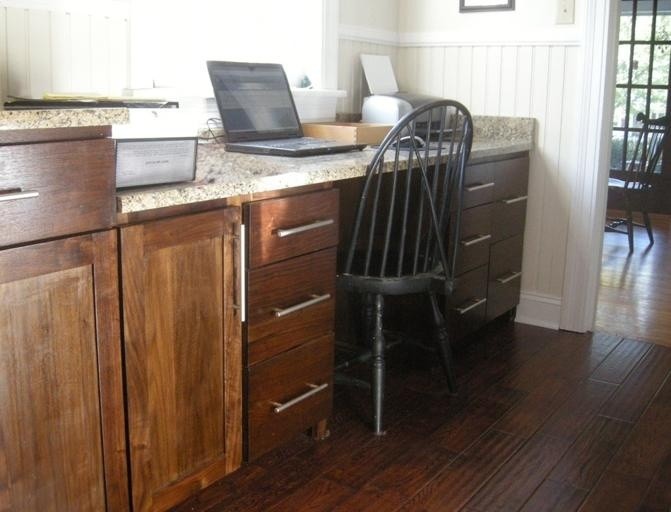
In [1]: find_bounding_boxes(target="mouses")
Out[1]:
[392,136,424,148]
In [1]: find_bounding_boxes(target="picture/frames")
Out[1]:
[459,0,515,13]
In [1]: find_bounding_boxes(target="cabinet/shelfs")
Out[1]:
[0,125,132,512]
[117,198,245,511]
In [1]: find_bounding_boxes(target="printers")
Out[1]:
[360,54,455,142]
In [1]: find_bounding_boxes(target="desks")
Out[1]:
[244,155,533,465]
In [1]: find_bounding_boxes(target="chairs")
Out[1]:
[605,116,670,254]
[337,100,473,436]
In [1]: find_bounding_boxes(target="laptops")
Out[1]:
[206,60,367,157]
